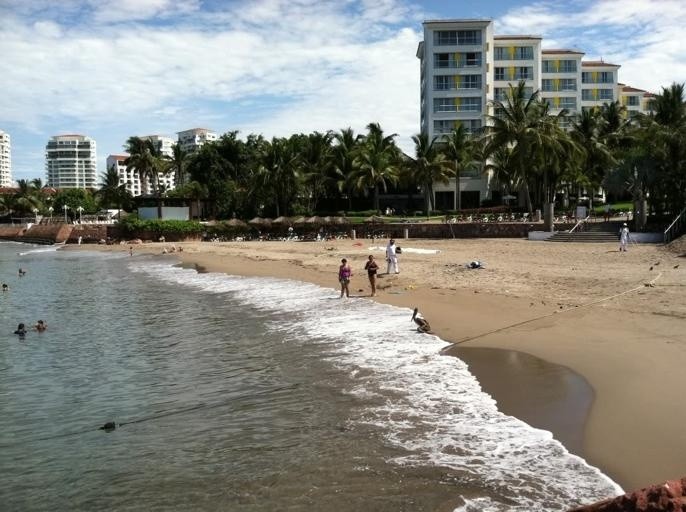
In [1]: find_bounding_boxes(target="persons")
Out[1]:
[129,247,133,257]
[2,284,8,291]
[162,245,183,255]
[365,255,379,297]
[386,240,400,274]
[18,268,26,275]
[34,320,46,331]
[604,203,611,223]
[14,324,27,335]
[619,223,630,253]
[338,259,351,298]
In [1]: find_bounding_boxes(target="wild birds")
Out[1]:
[411,307,434,334]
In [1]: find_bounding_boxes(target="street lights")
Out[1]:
[32,208,39,218]
[76,206,84,223]
[49,207,54,217]
[62,204,70,222]
